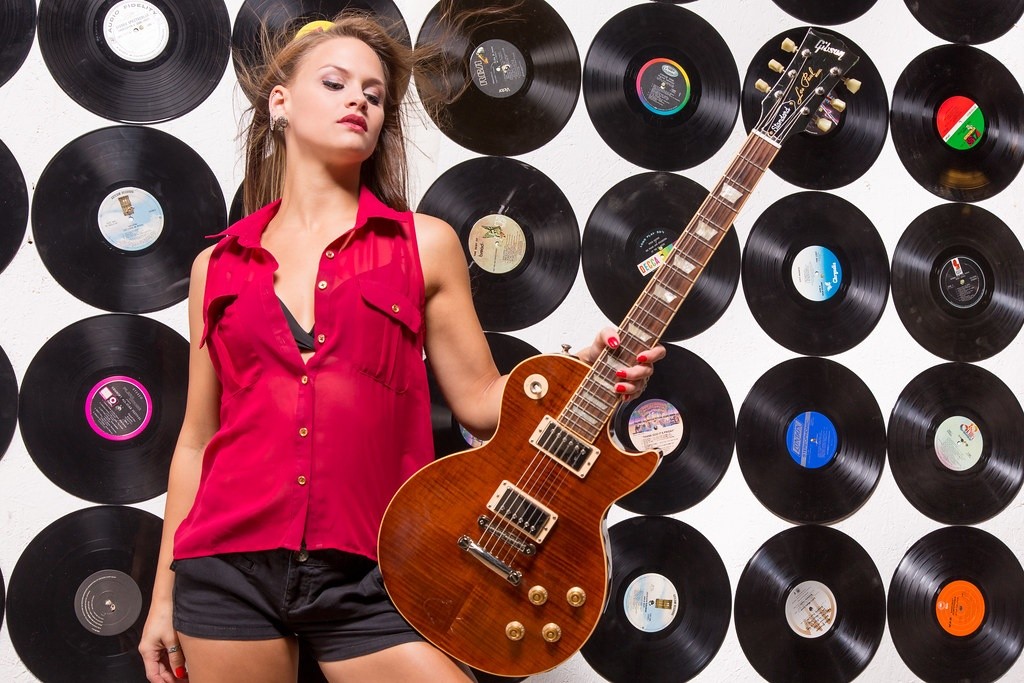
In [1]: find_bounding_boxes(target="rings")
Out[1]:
[167,646,181,653]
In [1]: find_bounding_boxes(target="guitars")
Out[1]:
[374,26,864,678]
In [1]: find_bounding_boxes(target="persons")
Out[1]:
[139,17,666,683]
